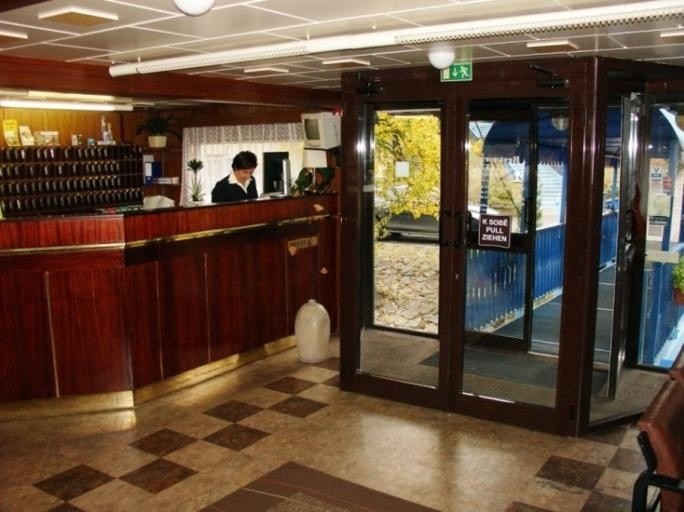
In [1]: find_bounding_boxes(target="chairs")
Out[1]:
[632,379,683,511]
[666,338,683,387]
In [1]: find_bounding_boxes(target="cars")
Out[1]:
[374,183,496,247]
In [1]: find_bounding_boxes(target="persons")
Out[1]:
[210,150,260,203]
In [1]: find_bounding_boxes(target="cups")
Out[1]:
[273,181,282,191]
[88,137,96,146]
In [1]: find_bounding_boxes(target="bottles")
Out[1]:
[78,134,82,145]
[101,116,113,144]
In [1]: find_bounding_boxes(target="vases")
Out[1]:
[295,298,332,364]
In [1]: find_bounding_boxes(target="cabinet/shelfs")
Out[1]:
[0,142,145,219]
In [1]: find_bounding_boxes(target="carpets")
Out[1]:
[421,345,608,399]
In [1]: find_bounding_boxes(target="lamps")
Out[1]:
[171,1,215,17]
[426,51,455,70]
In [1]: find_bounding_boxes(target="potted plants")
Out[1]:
[135,112,181,149]
[670,255,683,305]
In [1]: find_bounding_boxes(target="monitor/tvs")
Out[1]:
[263,151,291,199]
[301,112,341,150]
